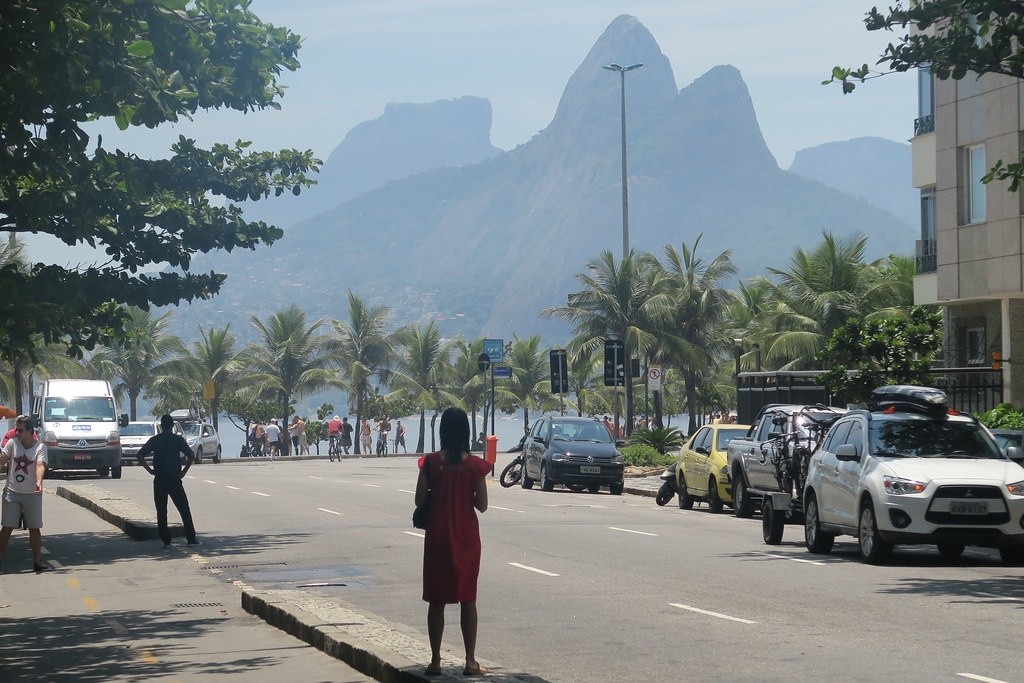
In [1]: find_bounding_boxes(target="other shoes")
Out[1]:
[188,539,202,546]
[270,457,277,461]
[464,661,488,674]
[424,664,441,675]
[162,538,171,546]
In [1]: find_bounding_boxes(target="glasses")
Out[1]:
[15,428,23,433]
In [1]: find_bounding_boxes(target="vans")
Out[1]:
[31,378,130,479]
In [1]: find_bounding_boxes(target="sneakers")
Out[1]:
[34,563,51,572]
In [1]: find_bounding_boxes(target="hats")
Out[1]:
[603,416,608,419]
[271,419,277,421]
[333,415,340,421]
[344,417,348,421]
[295,415,301,420]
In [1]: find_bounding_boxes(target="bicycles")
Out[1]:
[328,430,343,462]
[373,427,388,457]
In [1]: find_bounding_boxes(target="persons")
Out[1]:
[373,414,391,454]
[0,415,48,574]
[633,414,655,430]
[359,418,372,454]
[604,416,623,439]
[395,421,407,453]
[328,416,345,454]
[413,407,492,674]
[137,415,202,546]
[478,432,484,444]
[251,419,265,456]
[282,416,309,456]
[265,418,283,460]
[712,411,730,424]
[341,417,353,454]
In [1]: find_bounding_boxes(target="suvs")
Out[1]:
[169,408,222,464]
[801,382,1024,565]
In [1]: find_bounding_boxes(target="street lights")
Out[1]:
[601,62,644,441]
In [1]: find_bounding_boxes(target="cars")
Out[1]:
[946,428,1024,466]
[674,423,757,513]
[520,413,626,495]
[117,419,188,467]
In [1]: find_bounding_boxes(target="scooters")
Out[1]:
[655,433,687,506]
[500,417,530,488]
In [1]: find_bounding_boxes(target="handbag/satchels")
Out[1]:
[240,445,249,457]
[249,428,256,439]
[412,489,432,529]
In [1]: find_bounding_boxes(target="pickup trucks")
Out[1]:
[724,403,867,519]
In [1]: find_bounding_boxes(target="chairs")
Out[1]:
[553,426,562,437]
[579,430,591,440]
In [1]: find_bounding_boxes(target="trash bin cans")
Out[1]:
[485,435,497,465]
